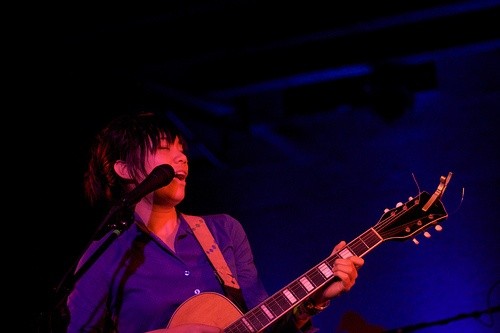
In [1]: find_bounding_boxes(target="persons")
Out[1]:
[65,104,364,333]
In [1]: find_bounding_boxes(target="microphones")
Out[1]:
[118,164,175,204]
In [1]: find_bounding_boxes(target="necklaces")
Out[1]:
[144,217,180,243]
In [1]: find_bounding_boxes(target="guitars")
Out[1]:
[168,172,454,333]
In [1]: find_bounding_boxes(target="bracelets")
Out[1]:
[296,296,332,320]
